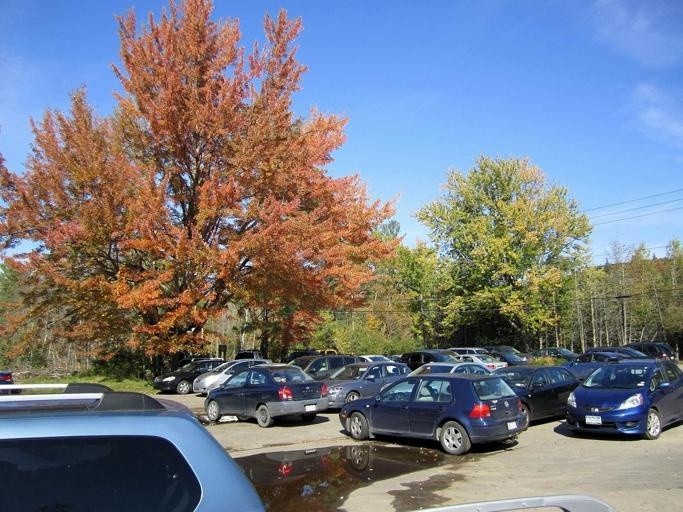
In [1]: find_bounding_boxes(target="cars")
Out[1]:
[153,349,413,428]
[0,383,266,512]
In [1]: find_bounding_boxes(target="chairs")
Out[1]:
[419,385,434,402]
